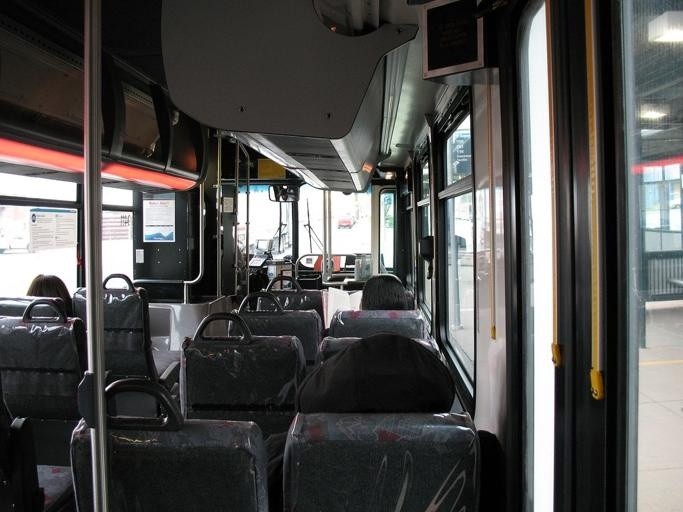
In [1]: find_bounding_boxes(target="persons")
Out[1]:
[24,275,86,366]
[357,272,413,311]
[207,211,245,278]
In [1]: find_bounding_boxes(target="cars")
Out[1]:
[0,232,36,253]
[338,219,352,229]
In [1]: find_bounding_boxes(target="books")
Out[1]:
[320,288,364,332]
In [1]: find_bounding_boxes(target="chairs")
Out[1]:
[230,291,324,363]
[282,411,479,511]
[0,299,86,467]
[329,310,427,339]
[2,390,74,510]
[69,369,269,510]
[315,335,441,374]
[1,294,64,317]
[72,272,179,395]
[256,276,326,326]
[178,312,308,442]
